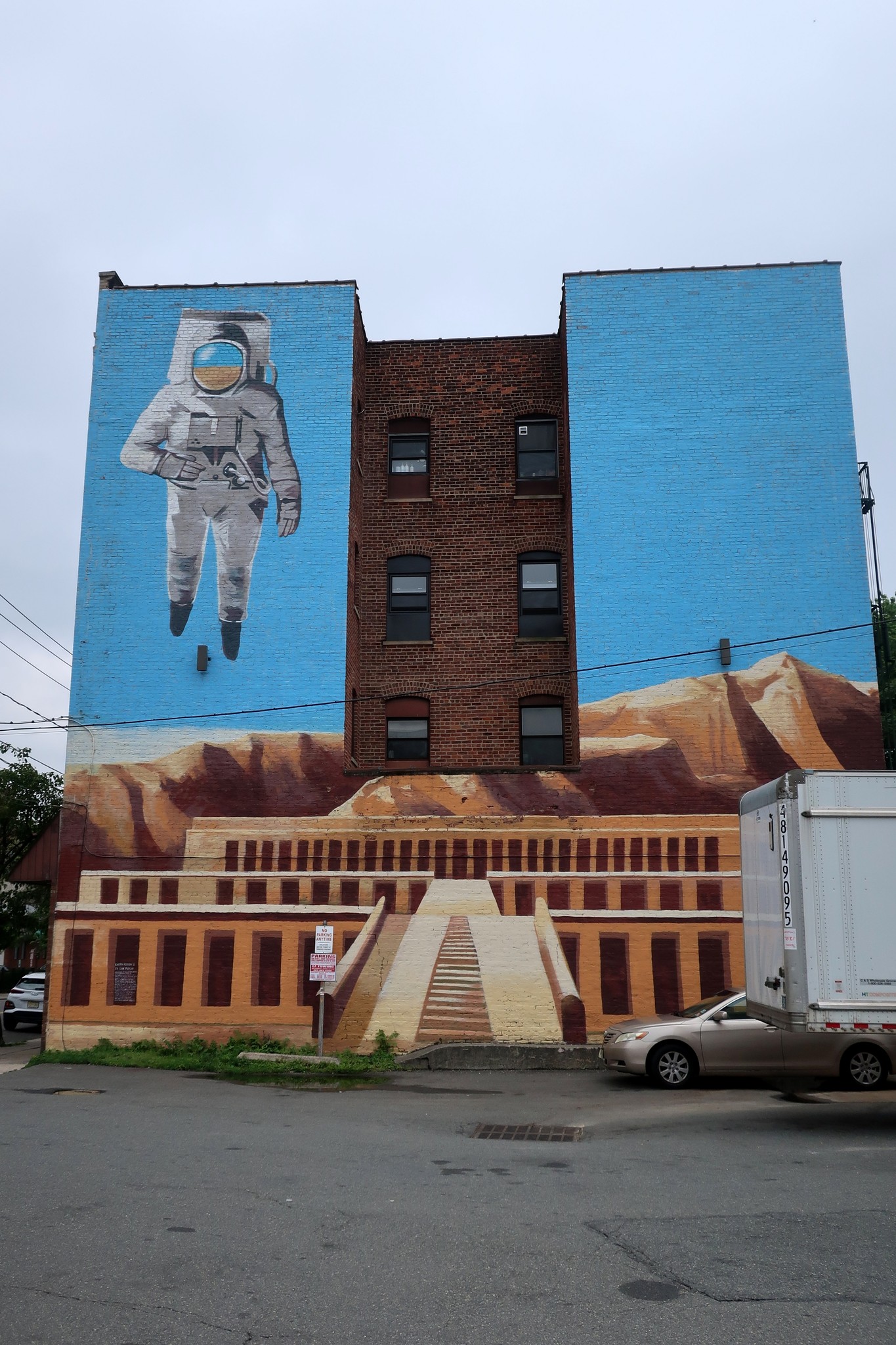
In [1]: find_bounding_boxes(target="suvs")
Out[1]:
[3,971,46,1033]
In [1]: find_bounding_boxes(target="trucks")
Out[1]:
[736,763,896,1038]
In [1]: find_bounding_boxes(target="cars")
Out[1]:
[598,986,896,1092]
